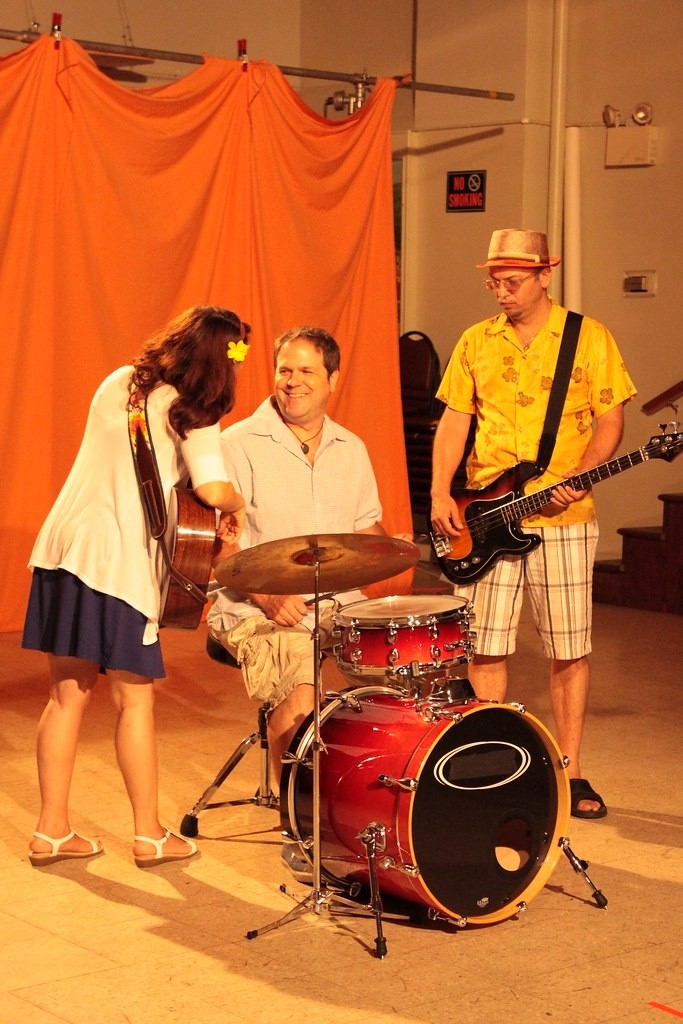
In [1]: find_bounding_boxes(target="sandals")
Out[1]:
[28,828,104,866]
[133,825,199,868]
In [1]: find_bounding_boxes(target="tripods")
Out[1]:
[247,554,371,940]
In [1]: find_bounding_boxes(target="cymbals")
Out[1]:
[214,533,423,596]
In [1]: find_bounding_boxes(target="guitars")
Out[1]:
[158,485,217,630]
[425,420,683,585]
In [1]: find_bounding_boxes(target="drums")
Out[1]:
[278,683,571,924]
[331,594,477,677]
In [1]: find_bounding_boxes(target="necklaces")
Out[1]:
[281,414,323,455]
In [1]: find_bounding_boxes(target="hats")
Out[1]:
[474,229,561,269]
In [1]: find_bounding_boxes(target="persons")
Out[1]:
[20,306,248,868]
[431,229,637,818]
[206,325,416,883]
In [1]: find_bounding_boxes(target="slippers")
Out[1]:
[567,778,608,819]
